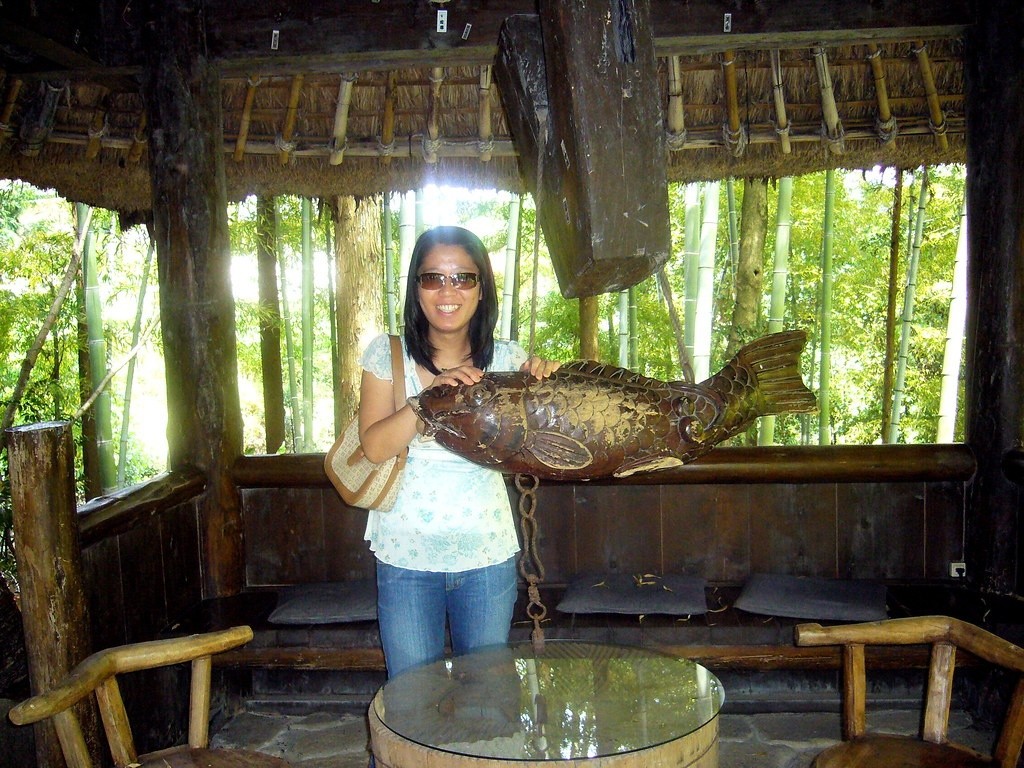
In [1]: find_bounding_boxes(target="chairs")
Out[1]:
[8,625,292,767]
[795,616,1023,768]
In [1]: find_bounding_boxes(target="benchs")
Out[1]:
[170,588,978,672]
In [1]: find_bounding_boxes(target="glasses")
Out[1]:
[416,272,482,290]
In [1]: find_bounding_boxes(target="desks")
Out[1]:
[368,638,726,768]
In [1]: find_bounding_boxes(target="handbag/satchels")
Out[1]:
[323,334,409,512]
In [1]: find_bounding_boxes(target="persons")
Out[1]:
[358,225,561,681]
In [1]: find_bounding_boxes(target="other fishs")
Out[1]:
[405,329,821,482]
